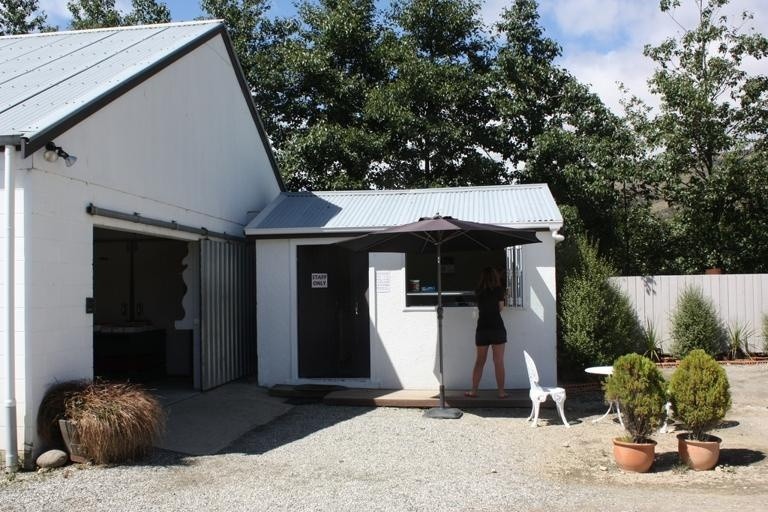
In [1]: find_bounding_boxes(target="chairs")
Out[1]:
[523,350,570,428]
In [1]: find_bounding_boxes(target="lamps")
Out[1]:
[44,142,76,168]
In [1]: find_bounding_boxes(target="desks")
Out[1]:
[584,366,625,429]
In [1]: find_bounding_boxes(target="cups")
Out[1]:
[409,279,421,292]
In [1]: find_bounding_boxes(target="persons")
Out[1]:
[464,265,513,398]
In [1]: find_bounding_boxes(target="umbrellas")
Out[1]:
[326,212,542,408]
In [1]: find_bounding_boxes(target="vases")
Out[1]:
[58,417,143,465]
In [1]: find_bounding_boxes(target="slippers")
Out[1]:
[498,392,512,400]
[463,390,478,398]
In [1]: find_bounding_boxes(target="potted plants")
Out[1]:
[667,349,731,470]
[601,353,665,473]
